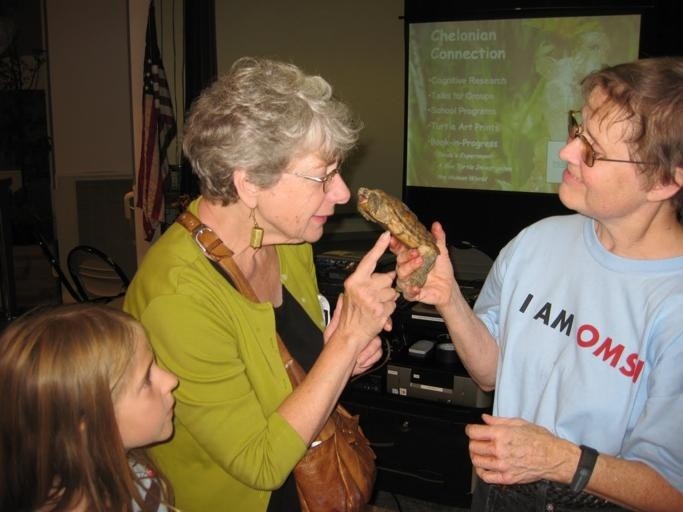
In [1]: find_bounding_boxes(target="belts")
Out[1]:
[513,484,620,510]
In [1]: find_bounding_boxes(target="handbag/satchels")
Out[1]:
[293,402,377,512]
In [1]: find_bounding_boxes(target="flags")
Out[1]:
[135,0,177,242]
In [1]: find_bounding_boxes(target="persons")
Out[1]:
[389,58,683,512]
[123,58,401,512]
[0,301,180,512]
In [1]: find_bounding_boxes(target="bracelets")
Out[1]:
[570,444,599,497]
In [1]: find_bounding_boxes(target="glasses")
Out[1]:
[285,169,340,193]
[568,110,674,168]
